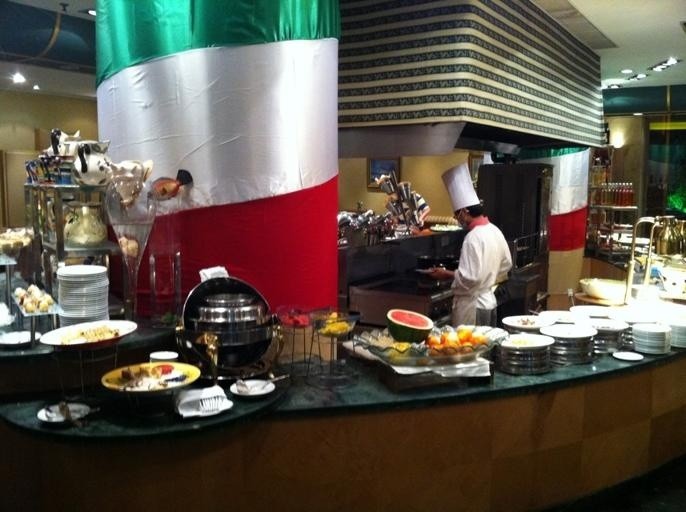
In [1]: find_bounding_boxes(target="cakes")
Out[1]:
[13,282,55,315]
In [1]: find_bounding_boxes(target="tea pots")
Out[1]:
[30,194,108,246]
[654,215,686,259]
[45,125,115,189]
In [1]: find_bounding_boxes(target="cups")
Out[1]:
[148,350,178,362]
[377,174,424,227]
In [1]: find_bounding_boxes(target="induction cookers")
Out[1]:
[367,278,463,298]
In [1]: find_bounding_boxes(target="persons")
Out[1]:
[429,160,513,328]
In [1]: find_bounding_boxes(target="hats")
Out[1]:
[441,163,480,213]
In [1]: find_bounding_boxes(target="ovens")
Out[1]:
[424,292,454,329]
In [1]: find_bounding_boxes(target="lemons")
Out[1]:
[387,338,412,363]
[316,312,351,336]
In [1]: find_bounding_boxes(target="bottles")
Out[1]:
[598,181,637,208]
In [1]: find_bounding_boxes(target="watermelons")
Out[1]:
[385,306,435,346]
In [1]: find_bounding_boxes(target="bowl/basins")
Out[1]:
[353,324,508,365]
[580,276,627,299]
[310,307,361,336]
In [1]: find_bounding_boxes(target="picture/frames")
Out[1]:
[367,158,401,193]
[468,154,484,190]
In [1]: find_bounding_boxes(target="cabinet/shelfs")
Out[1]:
[587,204,639,264]
[23,182,138,326]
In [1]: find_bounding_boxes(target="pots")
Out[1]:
[415,254,461,269]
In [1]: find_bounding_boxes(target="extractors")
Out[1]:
[451,121,577,159]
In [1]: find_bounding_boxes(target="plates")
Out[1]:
[430,224,462,233]
[39,317,138,346]
[10,287,56,319]
[498,303,686,376]
[35,400,87,425]
[229,376,280,398]
[0,329,40,349]
[54,263,110,328]
[100,360,202,393]
